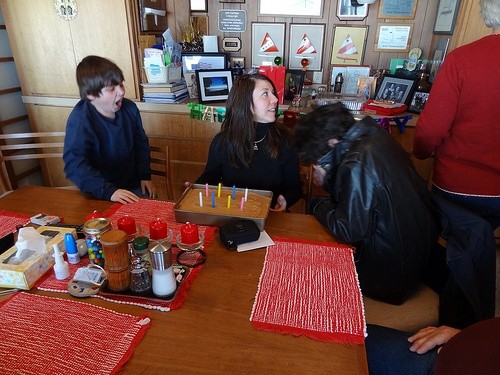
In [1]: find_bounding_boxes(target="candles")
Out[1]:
[180,221,199,244]
[199,183,248,210]
[118,216,137,235]
[86,210,105,222]
[150,217,167,240]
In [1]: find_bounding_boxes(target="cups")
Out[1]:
[127,225,143,244]
[145,228,173,245]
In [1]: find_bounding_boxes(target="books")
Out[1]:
[141,80,189,104]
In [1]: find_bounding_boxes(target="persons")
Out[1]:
[396,61,409,74]
[62,55,155,205]
[413,0,500,328]
[364,317,500,375]
[188,74,198,96]
[185,75,304,213]
[303,102,440,305]
[289,77,297,95]
[381,83,403,103]
[413,93,426,110]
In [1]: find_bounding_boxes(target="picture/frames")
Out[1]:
[257,0,326,18]
[432,0,461,35]
[284,70,304,100]
[189,0,208,12]
[374,0,418,52]
[373,73,419,104]
[250,21,327,71]
[181,52,233,103]
[329,0,370,66]
[328,64,372,95]
[407,91,430,114]
[390,58,428,74]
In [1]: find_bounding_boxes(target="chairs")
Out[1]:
[148,145,174,201]
[362,190,495,333]
[0,131,81,197]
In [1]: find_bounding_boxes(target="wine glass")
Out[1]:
[176,234,206,267]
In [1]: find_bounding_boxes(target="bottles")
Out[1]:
[53,217,177,298]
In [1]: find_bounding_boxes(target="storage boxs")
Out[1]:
[30,212,63,226]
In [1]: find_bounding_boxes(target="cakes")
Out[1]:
[177,189,270,218]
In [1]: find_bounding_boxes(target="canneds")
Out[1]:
[83,217,112,268]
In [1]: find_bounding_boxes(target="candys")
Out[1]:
[86,234,105,268]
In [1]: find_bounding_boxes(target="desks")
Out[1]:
[0,184,369,374]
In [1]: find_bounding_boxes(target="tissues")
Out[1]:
[0,225,78,291]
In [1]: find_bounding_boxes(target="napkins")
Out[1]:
[237,229,275,252]
